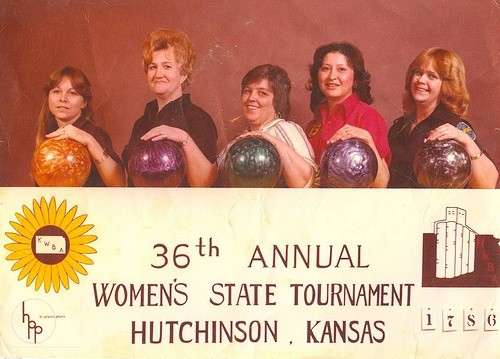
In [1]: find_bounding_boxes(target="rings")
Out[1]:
[63,129,67,134]
[346,130,350,136]
[161,134,167,141]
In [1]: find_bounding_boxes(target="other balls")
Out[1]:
[319,139,378,188]
[411,138,471,189]
[32,135,92,187]
[217,134,283,188]
[126,139,186,188]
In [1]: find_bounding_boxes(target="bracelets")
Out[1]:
[469,151,483,160]
[179,135,190,146]
[92,148,110,167]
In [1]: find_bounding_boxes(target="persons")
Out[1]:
[123,29,217,189]
[387,47,498,189]
[217,64,321,189]
[303,41,393,188]
[32,67,129,187]
